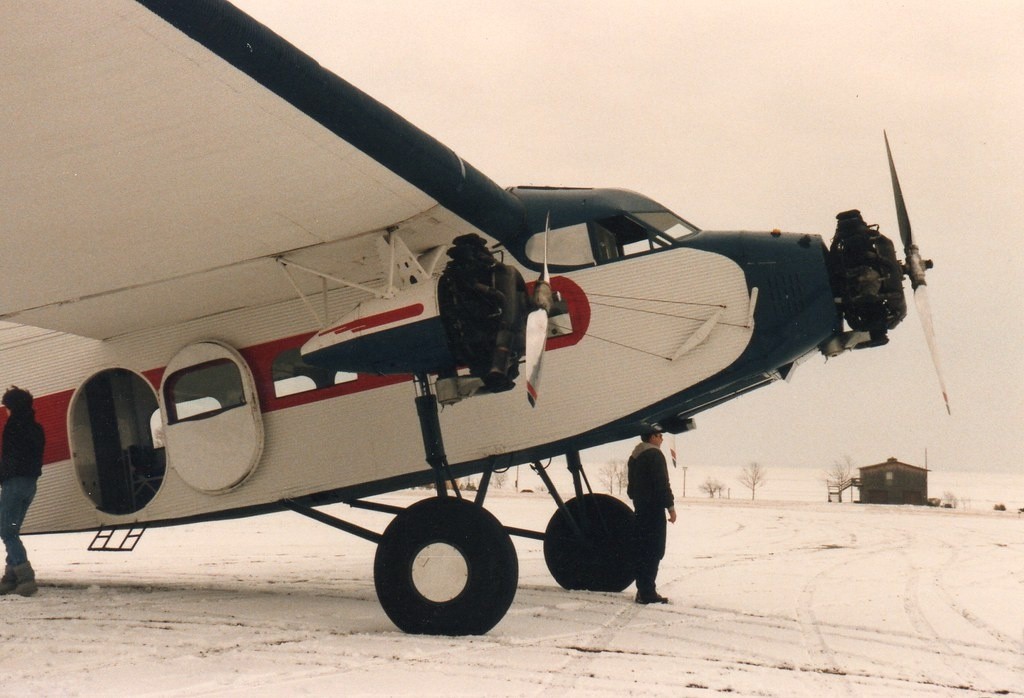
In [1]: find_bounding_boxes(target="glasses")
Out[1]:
[656,434,662,438]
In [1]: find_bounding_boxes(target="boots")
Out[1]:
[8,561,36,597]
[0,565,16,595]
[635,584,669,604]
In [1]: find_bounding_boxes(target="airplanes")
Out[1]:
[0,0,952,634]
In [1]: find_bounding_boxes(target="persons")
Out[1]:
[0,385,46,596]
[627,430,677,603]
